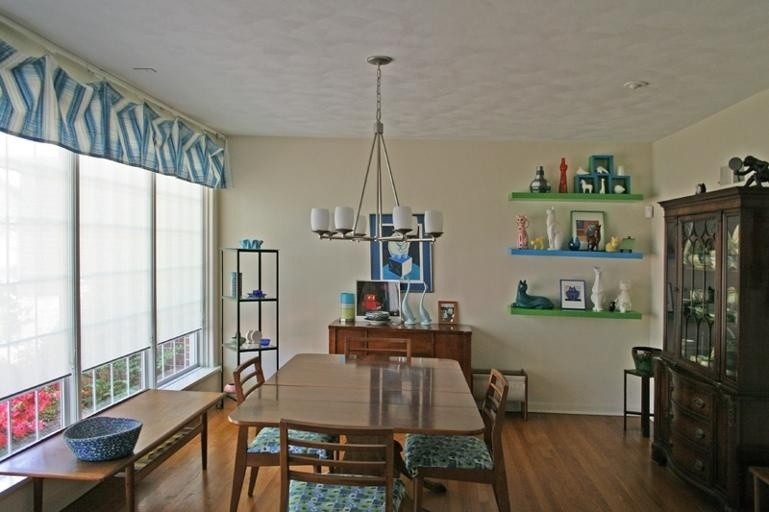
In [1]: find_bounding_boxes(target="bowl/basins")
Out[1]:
[232,338,246,346]
[260,339,270,346]
[367,311,389,319]
[63,417,144,461]
[240,240,263,249]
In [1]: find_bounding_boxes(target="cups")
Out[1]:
[253,290,262,294]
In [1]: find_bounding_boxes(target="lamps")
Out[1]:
[312,55,442,245]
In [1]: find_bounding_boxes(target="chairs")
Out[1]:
[344,338,411,358]
[231,356,340,509]
[621,347,663,438]
[405,369,510,509]
[279,418,405,509]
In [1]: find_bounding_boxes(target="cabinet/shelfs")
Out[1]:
[651,186,768,511]
[507,191,644,321]
[328,318,472,389]
[217,247,280,409]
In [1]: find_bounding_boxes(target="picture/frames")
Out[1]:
[560,278,587,311]
[369,213,433,294]
[437,301,458,325]
[571,209,607,250]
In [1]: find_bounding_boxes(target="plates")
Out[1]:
[364,318,391,325]
[247,294,267,297]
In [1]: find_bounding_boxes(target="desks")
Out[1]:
[231,353,485,503]
[1,390,228,510]
